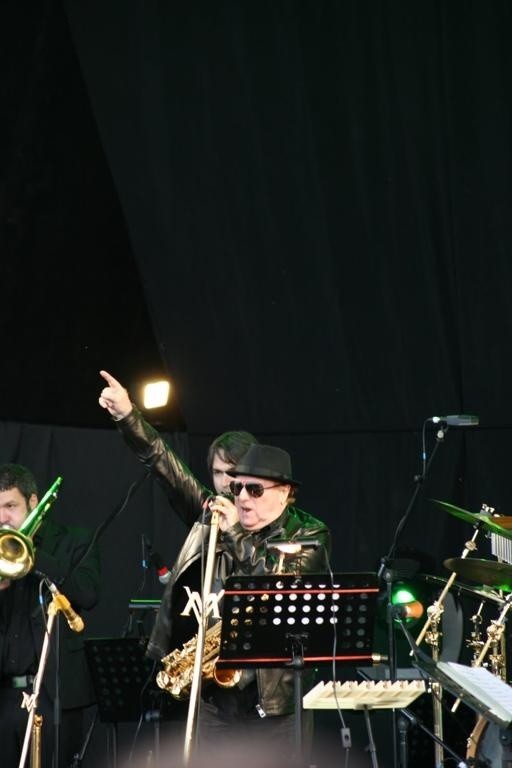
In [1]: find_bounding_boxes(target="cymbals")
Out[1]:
[425,573,506,603]
[433,497,512,540]
[444,558,511,591]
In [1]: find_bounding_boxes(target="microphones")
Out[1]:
[444,413,479,430]
[212,491,238,507]
[42,575,86,634]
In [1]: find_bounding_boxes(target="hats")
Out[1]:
[226,445,302,487]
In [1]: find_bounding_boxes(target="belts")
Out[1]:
[1,674,36,689]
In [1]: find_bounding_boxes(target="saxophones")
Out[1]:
[156,544,301,695]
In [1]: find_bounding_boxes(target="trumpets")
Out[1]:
[0,477,62,580]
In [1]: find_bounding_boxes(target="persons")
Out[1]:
[414,553,470,663]
[0,461,103,765]
[206,429,262,500]
[95,360,331,767]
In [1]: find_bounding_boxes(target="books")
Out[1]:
[435,658,512,723]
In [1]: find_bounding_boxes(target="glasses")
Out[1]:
[228,481,285,497]
